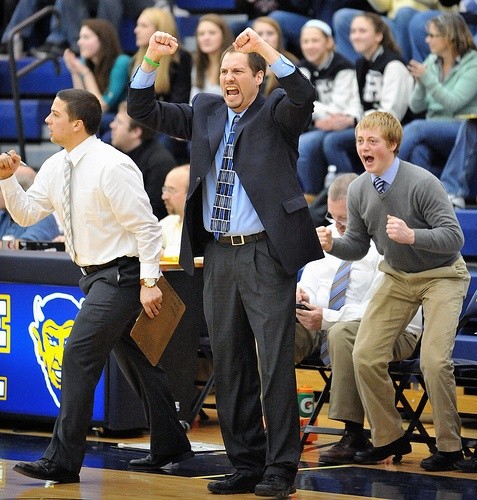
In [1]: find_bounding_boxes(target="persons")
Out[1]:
[0,87,197,485]
[158,163,213,423]
[315,109,470,472]
[126,26,326,496]
[0,0,477,249]
[293,172,422,461]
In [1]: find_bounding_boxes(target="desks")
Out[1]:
[0,248,205,437]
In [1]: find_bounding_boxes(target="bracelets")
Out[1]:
[143,55,160,68]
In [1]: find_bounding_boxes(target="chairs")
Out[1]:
[389,290,477,462]
[294,320,424,460]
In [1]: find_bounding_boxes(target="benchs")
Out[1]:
[0,0,477,379]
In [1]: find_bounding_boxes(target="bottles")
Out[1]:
[297,384,318,443]
[2,228,16,249]
[325,164,337,188]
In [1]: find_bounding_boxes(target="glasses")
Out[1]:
[324,211,348,228]
[425,32,445,39]
[161,186,189,200]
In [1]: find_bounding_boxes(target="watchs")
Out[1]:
[138,278,157,288]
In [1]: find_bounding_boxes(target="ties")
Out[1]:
[210,115,242,239]
[63,154,76,263]
[320,259,353,366]
[373,177,386,194]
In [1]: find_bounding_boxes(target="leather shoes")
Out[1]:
[13,459,81,483]
[420,452,466,471]
[257,475,296,496]
[354,438,412,463]
[319,435,373,463]
[208,470,260,494]
[128,448,195,468]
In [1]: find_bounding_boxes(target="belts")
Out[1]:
[210,232,262,245]
[81,256,135,276]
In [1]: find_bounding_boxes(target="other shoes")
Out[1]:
[448,193,465,210]
[30,45,59,59]
[0,40,28,57]
